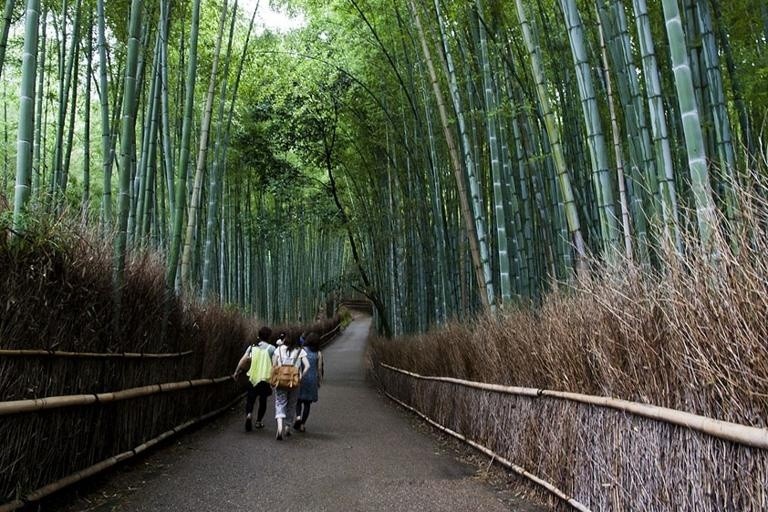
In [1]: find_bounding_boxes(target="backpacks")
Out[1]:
[270,345,301,388]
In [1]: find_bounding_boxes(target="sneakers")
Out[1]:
[245,412,305,440]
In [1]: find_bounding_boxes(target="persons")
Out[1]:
[275,332,286,347]
[292,332,322,433]
[232,325,276,432]
[299,331,307,346]
[271,328,311,442]
